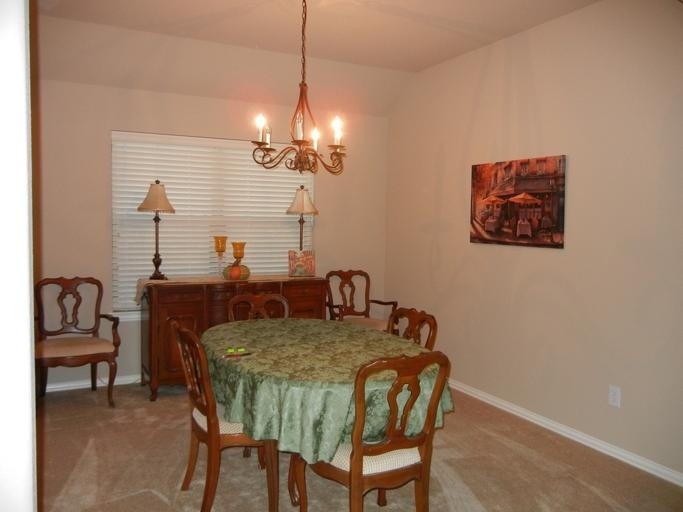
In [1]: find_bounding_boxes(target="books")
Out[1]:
[288,250,315,277]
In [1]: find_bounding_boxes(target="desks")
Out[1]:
[199,318,452,506]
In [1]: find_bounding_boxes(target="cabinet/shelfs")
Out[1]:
[139,277,326,401]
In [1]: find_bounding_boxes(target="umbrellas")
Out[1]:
[508,192,541,222]
[482,196,505,219]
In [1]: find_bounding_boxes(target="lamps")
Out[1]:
[136,180,177,281]
[212,2,347,264]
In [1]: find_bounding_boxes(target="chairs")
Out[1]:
[388,307,437,350]
[226,294,290,323]
[325,268,400,337]
[173,320,281,512]
[35,275,121,409]
[293,349,451,512]
